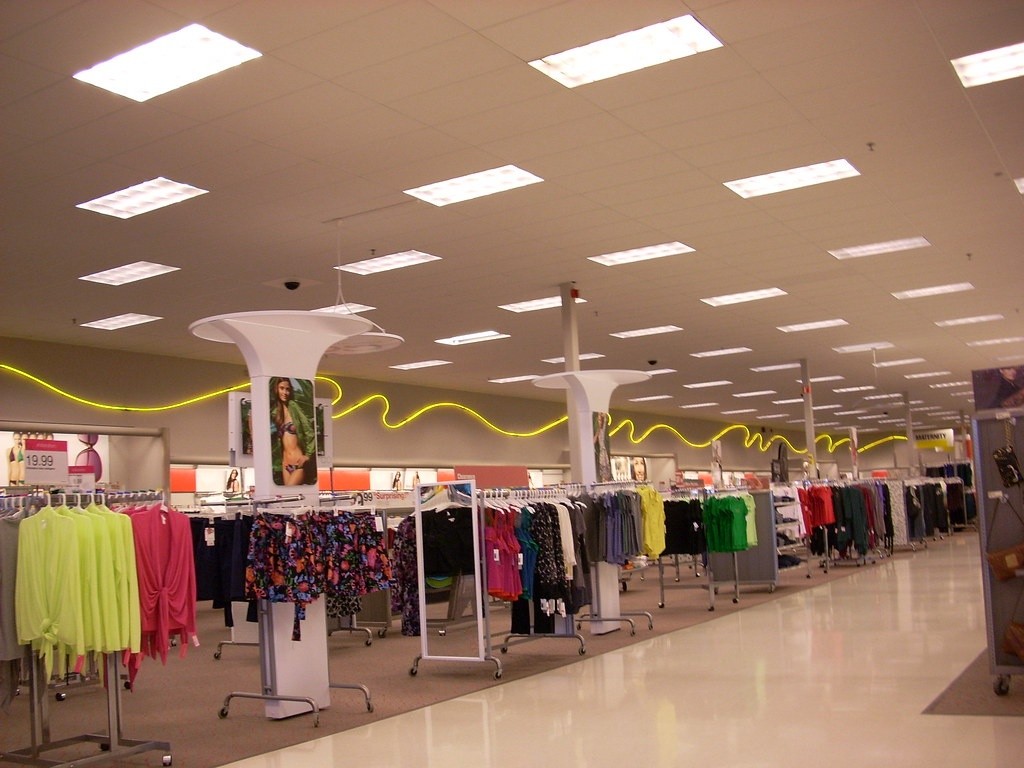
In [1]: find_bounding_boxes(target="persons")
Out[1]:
[990,366,1024,408]
[630,455,647,484]
[592,410,612,482]
[412,472,420,489]
[226,468,239,493]
[6,431,55,486]
[393,471,401,491]
[270,377,316,486]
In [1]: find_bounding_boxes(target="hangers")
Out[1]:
[805,476,963,491]
[411,481,752,515]
[173,500,376,519]
[0,484,168,513]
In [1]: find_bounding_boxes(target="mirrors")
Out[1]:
[416,479,485,662]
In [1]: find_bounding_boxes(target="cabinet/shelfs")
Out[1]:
[774,501,808,572]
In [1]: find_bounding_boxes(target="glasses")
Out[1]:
[75,432,101,482]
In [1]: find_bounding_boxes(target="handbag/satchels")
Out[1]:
[987,544,1024,582]
[1002,620,1024,664]
[992,418,1024,489]
